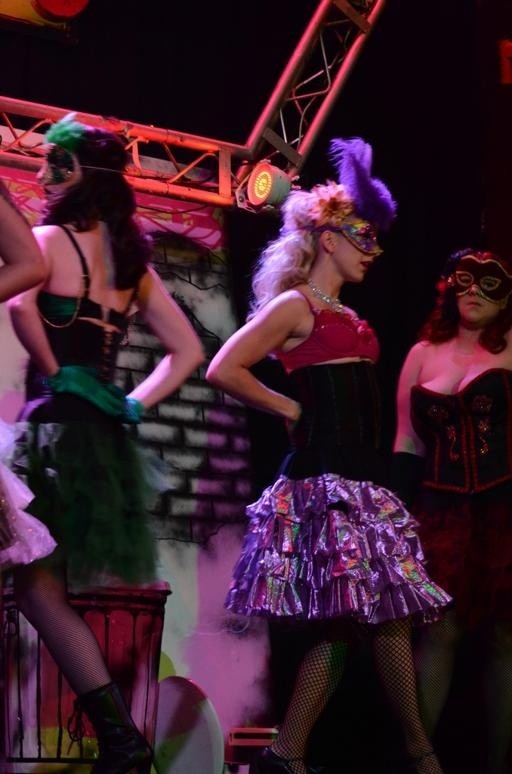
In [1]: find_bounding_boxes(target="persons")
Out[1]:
[8,123,205,773]
[205,184,454,774]
[393,248,511,743]
[1,177,58,571]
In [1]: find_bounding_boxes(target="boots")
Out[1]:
[68,681,156,774]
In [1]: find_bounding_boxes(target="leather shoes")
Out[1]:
[404,750,438,774]
[248,748,307,774]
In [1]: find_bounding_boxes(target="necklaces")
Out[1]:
[308,278,344,315]
